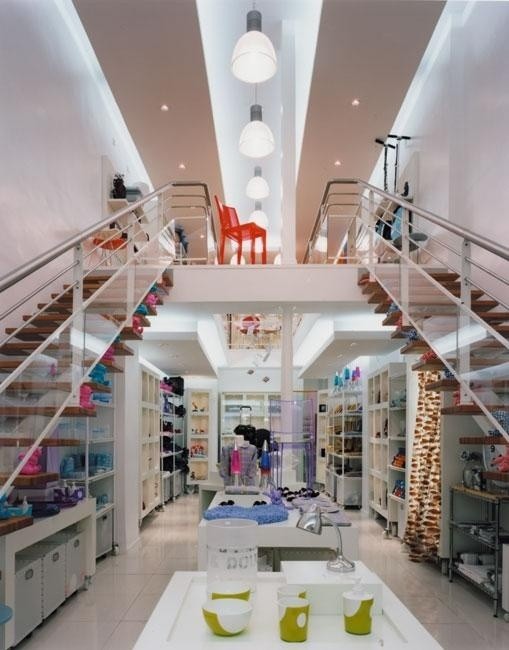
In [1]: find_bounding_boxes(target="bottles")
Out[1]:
[59,411,110,442]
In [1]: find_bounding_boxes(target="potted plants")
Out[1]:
[113,171,125,188]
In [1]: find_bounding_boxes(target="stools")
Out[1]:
[0,604,12,650]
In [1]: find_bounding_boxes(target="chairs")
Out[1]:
[374,209,428,266]
[213,197,269,265]
[87,227,126,268]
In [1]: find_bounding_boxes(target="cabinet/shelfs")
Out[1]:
[218,391,298,473]
[48,350,115,566]
[366,361,387,529]
[159,388,186,502]
[316,389,364,508]
[390,373,410,537]
[139,366,162,529]
[107,197,151,242]
[187,391,210,494]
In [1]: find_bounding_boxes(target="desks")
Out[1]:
[197,486,338,568]
[132,569,445,650]
[0,495,98,648]
[450,484,509,617]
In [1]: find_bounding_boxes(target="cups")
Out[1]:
[277,597,310,642]
[276,585,307,605]
[341,590,375,635]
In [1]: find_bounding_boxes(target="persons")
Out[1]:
[220,435,258,487]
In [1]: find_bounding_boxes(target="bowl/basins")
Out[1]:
[206,582,250,602]
[202,598,252,636]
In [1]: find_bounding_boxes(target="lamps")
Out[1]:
[239,80,276,159]
[297,504,356,573]
[247,165,270,202]
[229,0,277,84]
[249,199,270,231]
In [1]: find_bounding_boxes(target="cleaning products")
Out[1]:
[342,574,374,635]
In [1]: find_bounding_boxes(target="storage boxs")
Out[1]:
[95,510,112,558]
[13,530,85,646]
[111,188,146,207]
